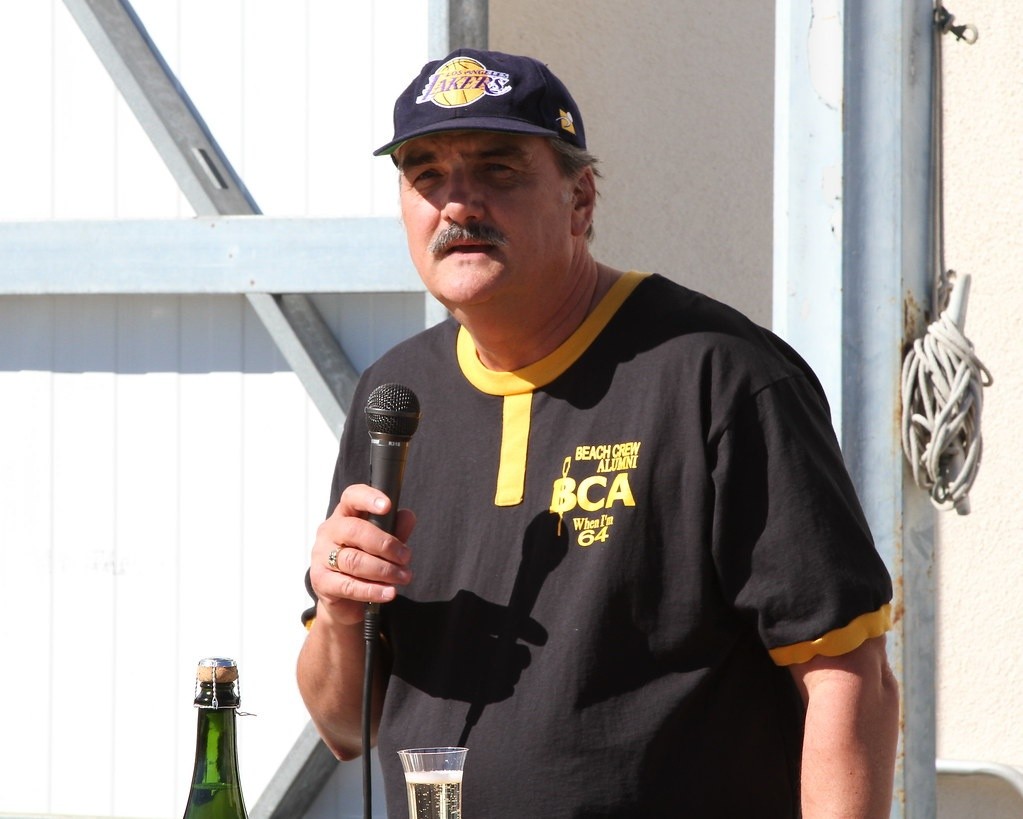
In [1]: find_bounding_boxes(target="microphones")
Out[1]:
[362,384,420,639]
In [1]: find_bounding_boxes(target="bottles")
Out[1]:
[182,657,250,819]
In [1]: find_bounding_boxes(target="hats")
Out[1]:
[373,49,587,154]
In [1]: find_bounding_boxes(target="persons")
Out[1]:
[295,49,898,819]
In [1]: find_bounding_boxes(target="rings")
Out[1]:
[327,544,345,571]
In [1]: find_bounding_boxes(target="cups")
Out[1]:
[398,745,467,819]
